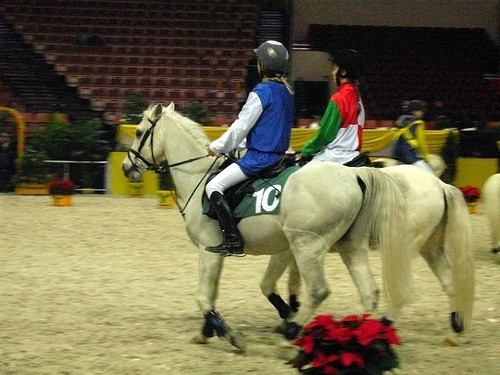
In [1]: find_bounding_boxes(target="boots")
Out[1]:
[205,191,244,255]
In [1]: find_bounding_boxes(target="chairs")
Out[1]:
[0,0,263,136]
[297,118,448,130]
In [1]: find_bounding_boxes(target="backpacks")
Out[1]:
[395,123,417,164]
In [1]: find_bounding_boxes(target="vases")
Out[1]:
[468,201,482,213]
[51,195,72,206]
[128,182,147,194]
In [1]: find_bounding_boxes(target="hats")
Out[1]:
[327,48,367,78]
[408,100,431,112]
[253,40,290,71]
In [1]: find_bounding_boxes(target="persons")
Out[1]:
[296,47,365,166]
[204,40,296,254]
[387,100,437,178]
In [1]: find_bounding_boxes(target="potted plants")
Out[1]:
[14,149,58,195]
[157,161,176,209]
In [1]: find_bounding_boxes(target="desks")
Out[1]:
[115,124,450,154]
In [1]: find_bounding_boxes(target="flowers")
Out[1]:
[47,179,76,196]
[284,312,403,375]
[460,186,481,201]
